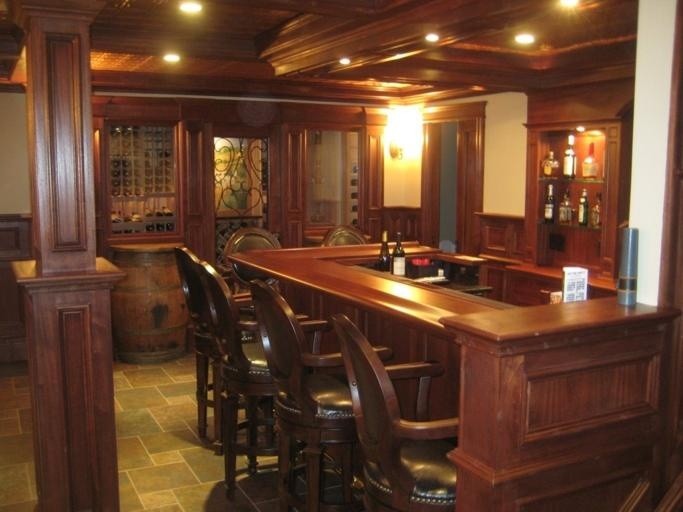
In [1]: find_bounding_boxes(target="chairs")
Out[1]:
[250,280,394,512]
[223,226,281,292]
[329,312,461,507]
[200,263,328,502]
[172,243,271,454]
[321,225,368,246]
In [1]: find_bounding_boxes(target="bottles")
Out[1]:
[592,192,603,228]
[544,184,555,224]
[563,135,576,177]
[619,227,636,304]
[579,189,588,225]
[541,151,561,176]
[559,189,572,224]
[378,231,391,271]
[582,142,600,180]
[391,231,405,276]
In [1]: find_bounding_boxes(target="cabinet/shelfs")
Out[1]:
[226,240,620,512]
[521,89,623,279]
